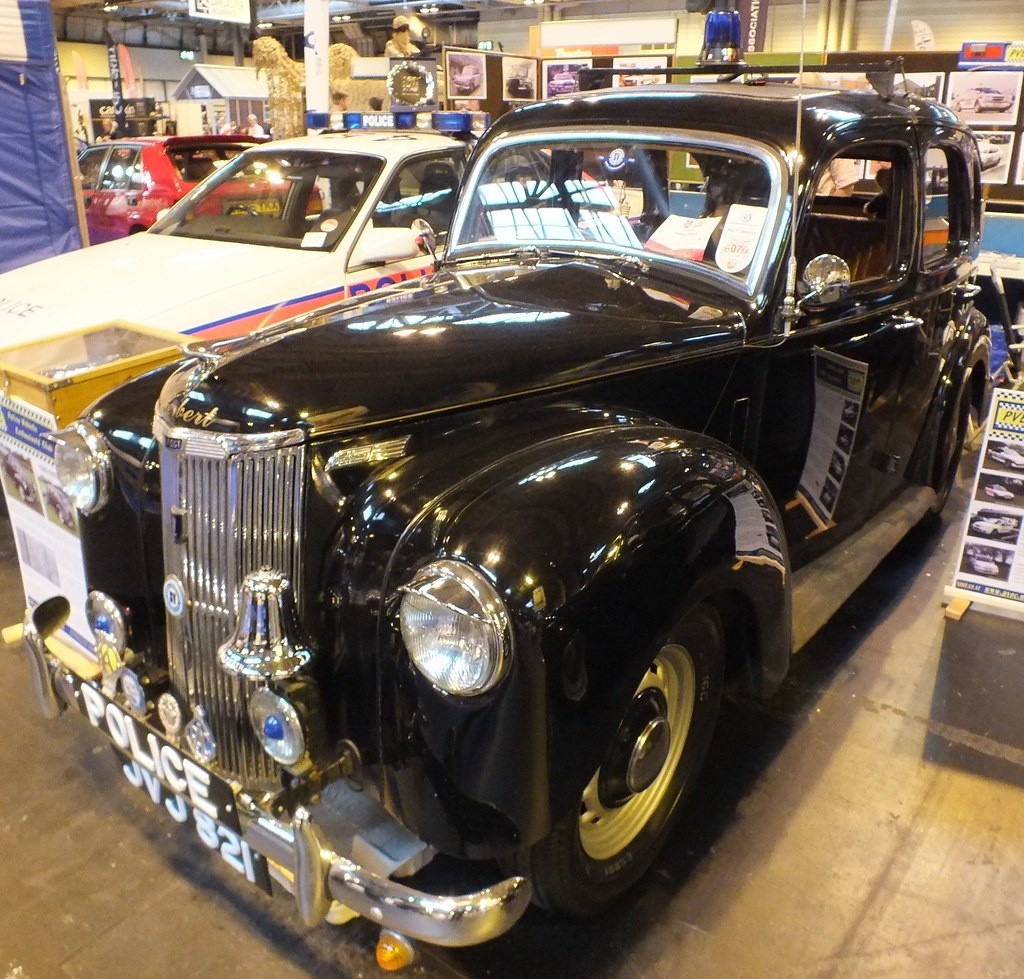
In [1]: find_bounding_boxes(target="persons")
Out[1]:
[76,112,265,150]
[330,93,383,112]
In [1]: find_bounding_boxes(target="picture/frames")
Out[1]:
[446,52,487,100]
[971,130,1016,185]
[1015,132,1024,184]
[541,59,593,99]
[690,74,745,83]
[501,56,538,102]
[612,57,668,87]
[768,75,799,83]
[946,71,1023,126]
[893,72,945,103]
[686,153,700,169]
[865,160,891,180]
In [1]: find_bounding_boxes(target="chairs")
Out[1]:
[399,161,459,245]
[185,157,212,181]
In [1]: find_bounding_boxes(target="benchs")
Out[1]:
[798,212,886,282]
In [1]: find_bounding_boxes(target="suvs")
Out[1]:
[26,12,995,979]
[449,64,484,95]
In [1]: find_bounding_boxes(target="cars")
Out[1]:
[948,86,1014,116]
[71,134,326,247]
[966,545,1005,577]
[0,108,480,481]
[547,72,582,97]
[966,130,1015,179]
[987,443,1024,472]
[971,517,1019,538]
[623,72,656,87]
[984,481,1016,502]
